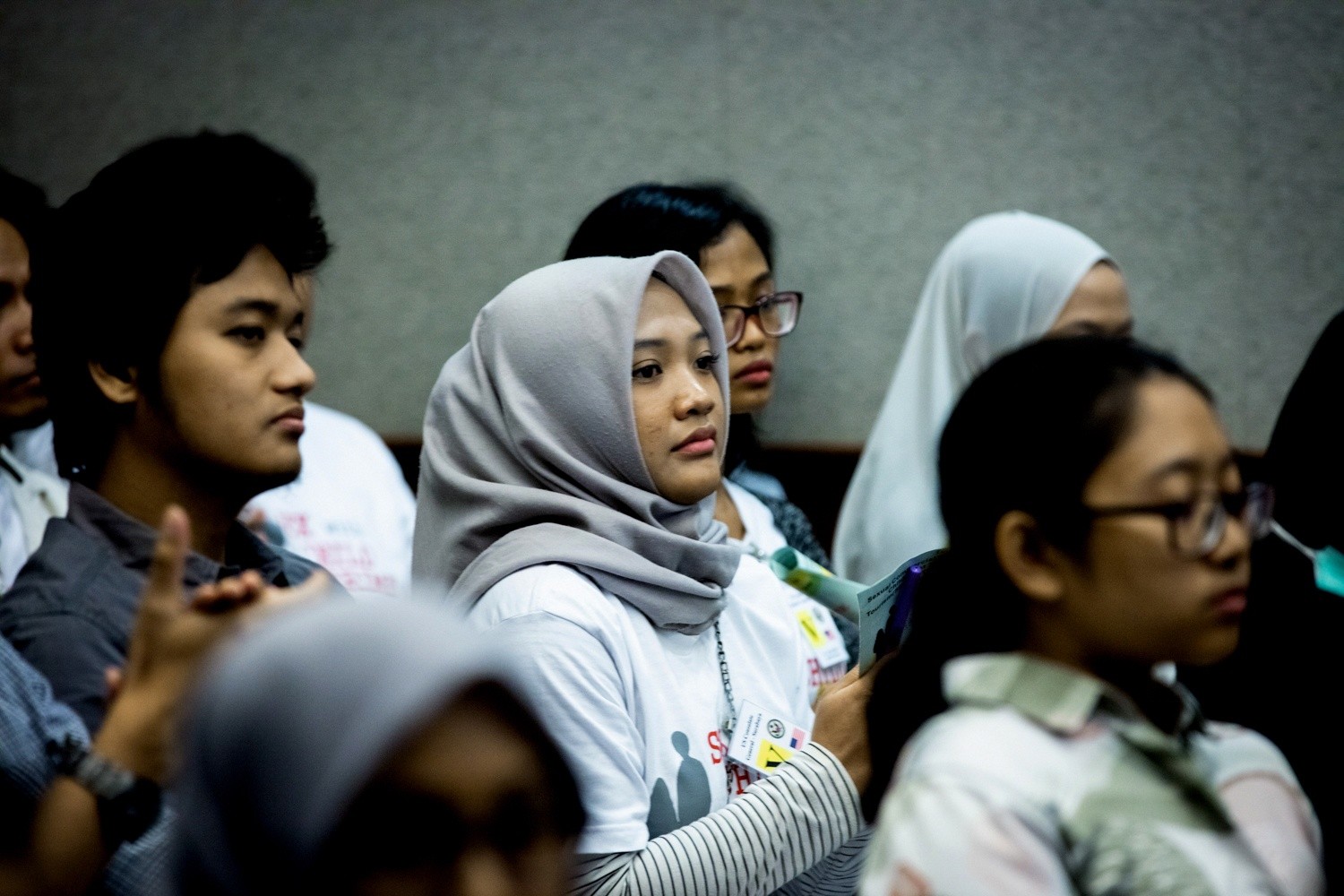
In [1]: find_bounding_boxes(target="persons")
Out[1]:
[175,590,591,895]
[563,181,827,574]
[835,209,1139,588]
[0,501,334,896]
[855,334,1323,894]
[0,167,70,592]
[1178,304,1343,896]
[0,131,359,741]
[418,248,899,895]
[27,275,419,599]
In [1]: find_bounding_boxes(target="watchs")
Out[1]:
[64,752,163,847]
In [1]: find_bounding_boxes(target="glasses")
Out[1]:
[720,288,805,350]
[1086,480,1278,560]
